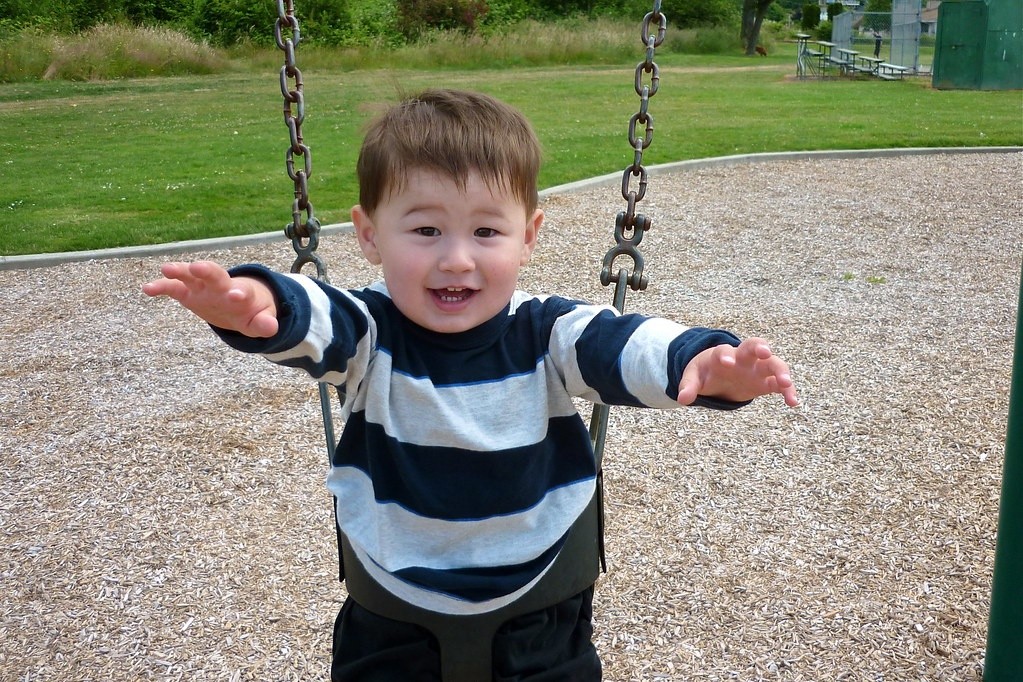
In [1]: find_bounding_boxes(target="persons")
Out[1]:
[872,30,883,59]
[141,77,799,681]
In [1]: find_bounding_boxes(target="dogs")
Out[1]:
[755,46,767,57]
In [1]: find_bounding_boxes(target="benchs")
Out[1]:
[795,33,909,82]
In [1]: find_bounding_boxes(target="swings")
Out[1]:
[267,0,664,679]
